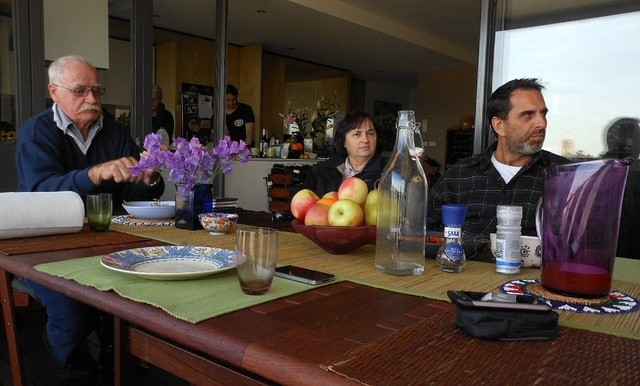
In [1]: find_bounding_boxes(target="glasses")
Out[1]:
[52,83,105,98]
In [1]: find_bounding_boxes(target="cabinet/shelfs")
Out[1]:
[447,125,474,169]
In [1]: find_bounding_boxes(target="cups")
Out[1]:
[86,193,113,231]
[535,156,634,299]
[236,227,278,294]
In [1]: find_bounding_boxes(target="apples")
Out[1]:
[364,187,391,213]
[327,199,364,227]
[366,201,400,226]
[322,192,338,201]
[304,203,328,226]
[337,177,368,208]
[290,189,321,220]
[316,198,337,207]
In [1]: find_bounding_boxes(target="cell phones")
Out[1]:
[274,265,335,287]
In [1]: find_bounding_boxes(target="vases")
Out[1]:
[304,139,315,155]
[174,182,213,230]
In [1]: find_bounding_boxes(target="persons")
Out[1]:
[421,158,441,191]
[16,55,165,381]
[409,77,577,236]
[1,122,16,144]
[287,109,392,204]
[209,84,255,149]
[603,117,640,174]
[151,85,174,145]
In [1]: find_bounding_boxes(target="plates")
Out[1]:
[385,228,491,261]
[100,245,246,281]
[122,201,175,219]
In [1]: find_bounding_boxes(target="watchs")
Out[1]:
[145,177,161,188]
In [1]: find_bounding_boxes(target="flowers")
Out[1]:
[281,94,345,137]
[131,133,252,197]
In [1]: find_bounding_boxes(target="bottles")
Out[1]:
[267,136,277,158]
[496,204,523,275]
[259,128,269,157]
[374,110,427,277]
[436,203,468,274]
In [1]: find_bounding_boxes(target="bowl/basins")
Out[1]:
[490,231,542,269]
[198,213,239,236]
[285,225,388,254]
